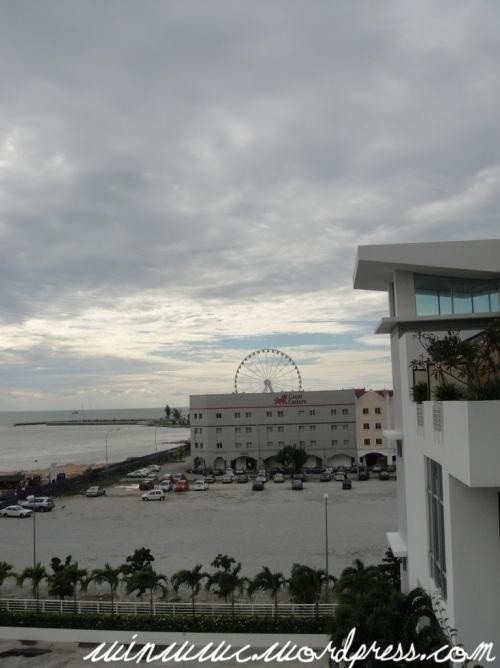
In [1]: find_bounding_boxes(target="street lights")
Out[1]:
[324,494,330,604]
[27,495,36,599]
[106,427,120,480]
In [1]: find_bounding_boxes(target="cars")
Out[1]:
[320,465,389,489]
[0,496,54,518]
[86,486,105,497]
[221,473,303,491]
[127,465,216,501]
